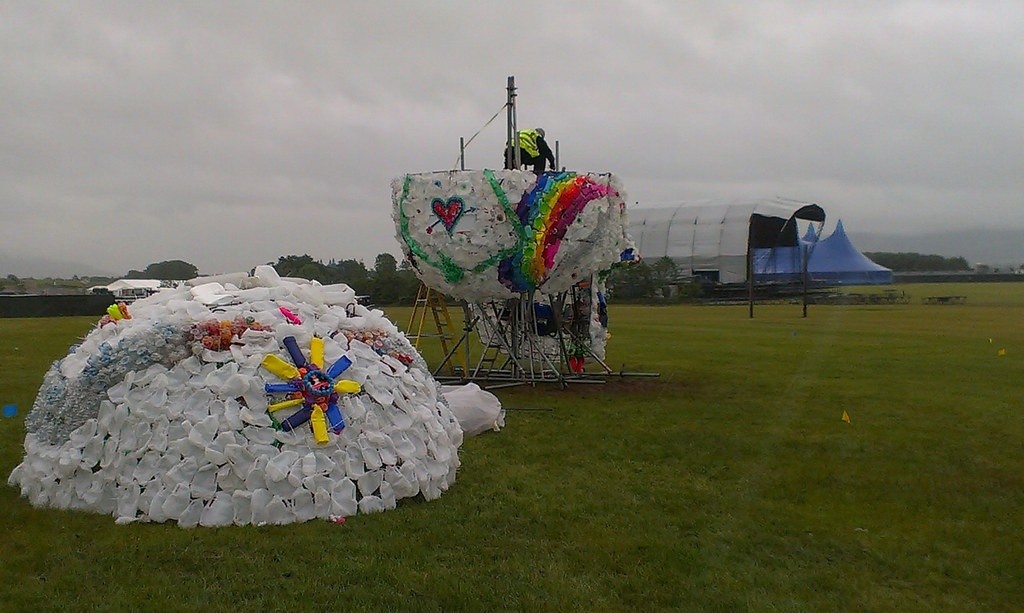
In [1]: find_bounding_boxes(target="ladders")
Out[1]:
[405,282,468,377]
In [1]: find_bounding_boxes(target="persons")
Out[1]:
[504,128,556,171]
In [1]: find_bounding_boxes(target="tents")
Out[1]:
[752,219,893,295]
